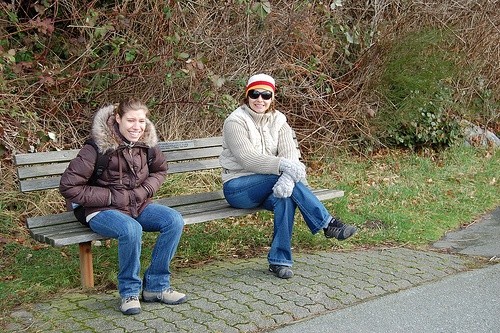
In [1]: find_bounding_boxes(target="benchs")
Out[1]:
[14,136,344,288]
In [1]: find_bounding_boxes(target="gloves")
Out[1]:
[272,172,294,198]
[279,158,307,183]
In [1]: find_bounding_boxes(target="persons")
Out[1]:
[59,96,187,314]
[219,74,356,278]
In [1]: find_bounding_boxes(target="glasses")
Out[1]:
[248,89,272,100]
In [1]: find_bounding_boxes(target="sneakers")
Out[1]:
[143,288,187,305]
[323,218,356,241]
[121,295,141,314]
[269,264,293,278]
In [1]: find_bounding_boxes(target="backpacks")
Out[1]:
[72,135,152,227]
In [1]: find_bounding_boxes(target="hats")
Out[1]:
[246,73,275,100]
